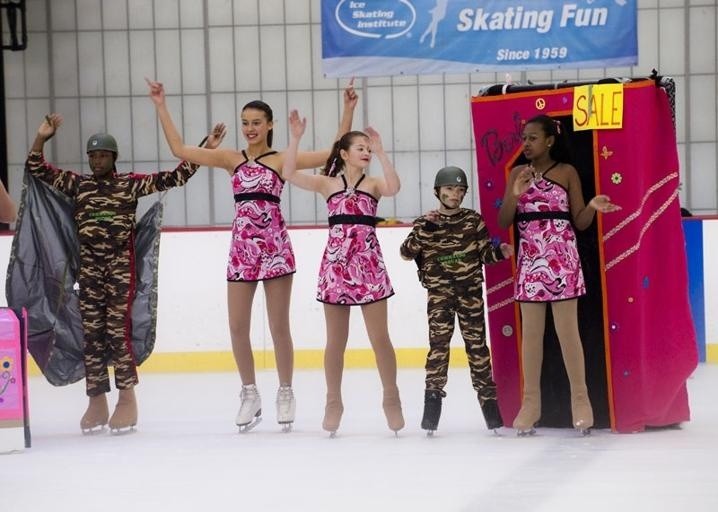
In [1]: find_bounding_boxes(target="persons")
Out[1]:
[399,165,518,430]
[25,110,230,430]
[143,73,359,426]
[497,112,626,432]
[0,180,18,225]
[281,106,406,431]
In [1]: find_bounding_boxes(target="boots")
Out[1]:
[108,388,138,429]
[512,389,543,431]
[275,384,297,423]
[480,397,505,430]
[79,393,110,430]
[570,384,594,432]
[234,383,263,428]
[322,391,345,431]
[421,387,442,431]
[381,387,406,432]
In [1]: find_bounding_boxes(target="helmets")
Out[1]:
[433,165,469,189]
[86,132,119,155]
